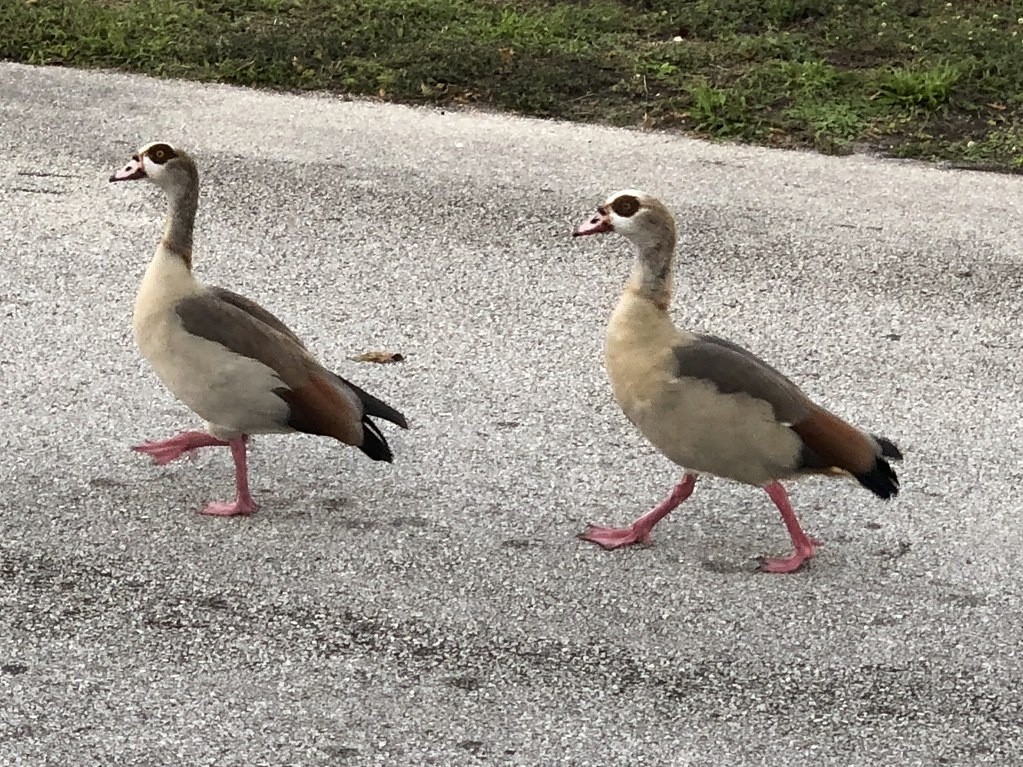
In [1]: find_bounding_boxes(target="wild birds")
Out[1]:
[108,141,409,517]
[569,189,905,574]
